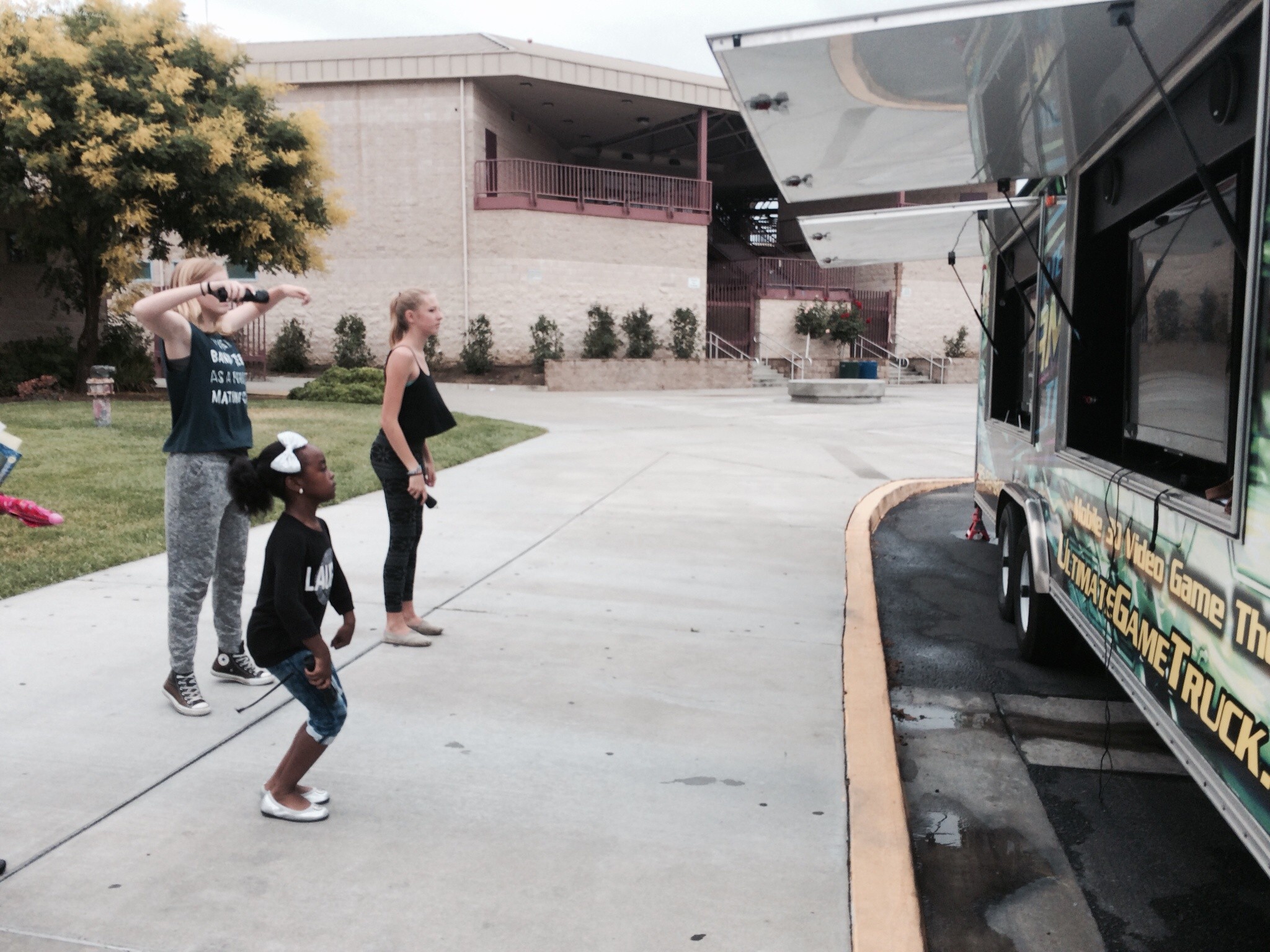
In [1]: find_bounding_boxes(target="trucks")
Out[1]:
[701,2,1270,879]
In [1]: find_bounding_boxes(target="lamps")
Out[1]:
[637,118,650,125]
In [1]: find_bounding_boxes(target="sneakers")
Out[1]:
[162,669,211,716]
[211,641,273,684]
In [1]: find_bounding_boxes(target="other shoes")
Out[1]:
[261,791,329,822]
[384,628,432,647]
[262,779,330,804]
[407,618,442,636]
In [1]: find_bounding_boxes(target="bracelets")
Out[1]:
[201,281,213,296]
[407,466,422,475]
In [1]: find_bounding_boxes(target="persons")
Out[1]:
[228,432,356,822]
[132,256,314,722]
[374,290,457,648]
